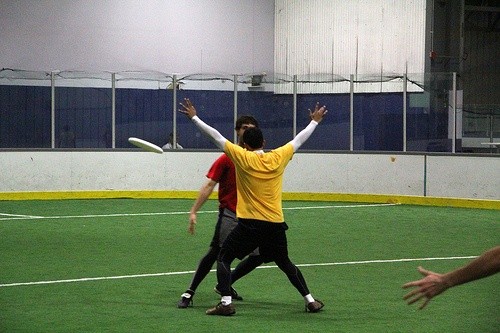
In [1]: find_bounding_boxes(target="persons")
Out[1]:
[178,99,328,315]
[178,115,263,308]
[403,246,500,309]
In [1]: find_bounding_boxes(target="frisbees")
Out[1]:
[128,137,164,155]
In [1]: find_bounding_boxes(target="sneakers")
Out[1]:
[305,298,325,313]
[177,289,194,308]
[214,283,243,301]
[205,301,235,316]
